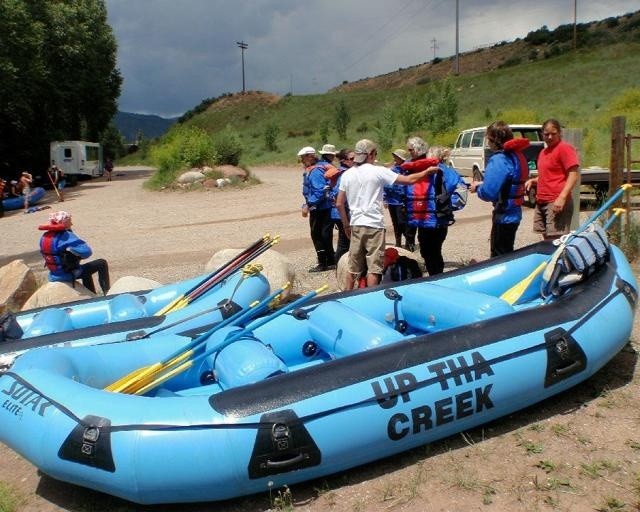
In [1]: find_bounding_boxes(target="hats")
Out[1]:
[318,143,340,155]
[392,148,408,161]
[352,138,377,163]
[297,146,316,155]
[49,211,73,230]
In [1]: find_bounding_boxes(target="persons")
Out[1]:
[9,180,20,198]
[468,121,530,259]
[296,136,465,292]
[37,211,110,296]
[20,170,32,209]
[52,165,66,201]
[525,119,580,240]
[105,159,114,181]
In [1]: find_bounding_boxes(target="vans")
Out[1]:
[443,124,552,189]
[49,140,104,179]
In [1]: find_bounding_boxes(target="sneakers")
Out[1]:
[309,264,327,273]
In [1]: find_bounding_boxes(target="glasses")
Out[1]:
[408,149,414,152]
[372,150,377,155]
[346,156,354,161]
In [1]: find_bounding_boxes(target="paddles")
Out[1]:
[133,286,332,396]
[122,281,290,394]
[113,301,258,393]
[154,234,281,316]
[499,183,633,305]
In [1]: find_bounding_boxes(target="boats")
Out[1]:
[2,268,274,378]
[1,232,639,506]
[1,184,46,212]
